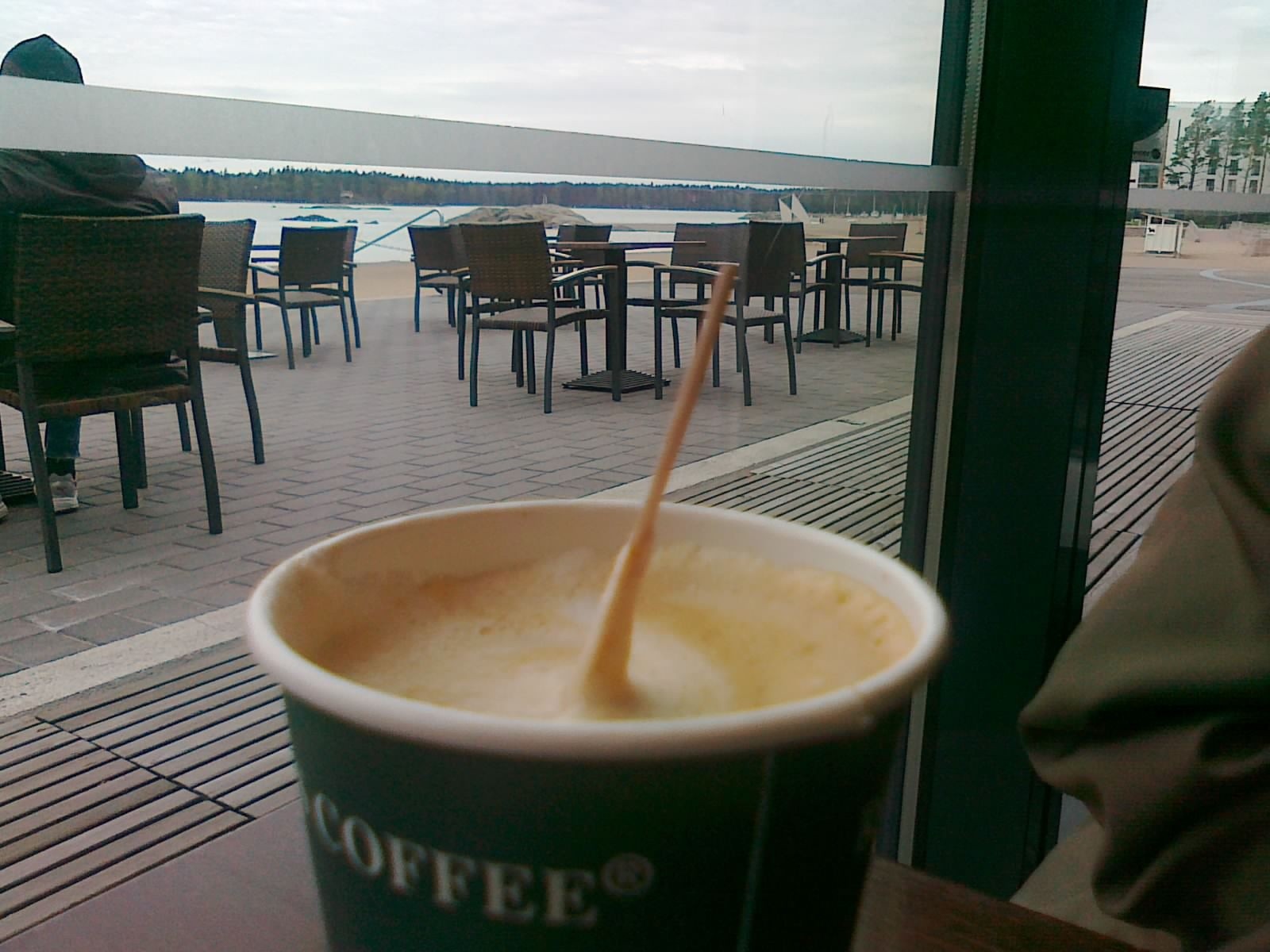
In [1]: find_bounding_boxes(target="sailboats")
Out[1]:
[778,193,813,225]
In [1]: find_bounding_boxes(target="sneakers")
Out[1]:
[35,471,79,513]
[0,502,8,521]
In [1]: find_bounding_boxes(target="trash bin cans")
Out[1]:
[1141,210,1187,252]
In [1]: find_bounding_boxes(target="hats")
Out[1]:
[1,34,84,85]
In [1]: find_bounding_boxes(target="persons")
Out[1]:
[1019,323,1269,952]
[0,34,180,519]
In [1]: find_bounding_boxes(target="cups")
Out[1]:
[243,498,949,952]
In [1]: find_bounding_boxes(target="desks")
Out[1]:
[792,235,897,345]
[245,242,284,359]
[548,240,706,395]
[0,793,1142,952]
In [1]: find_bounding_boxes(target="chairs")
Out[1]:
[0,211,932,576]
